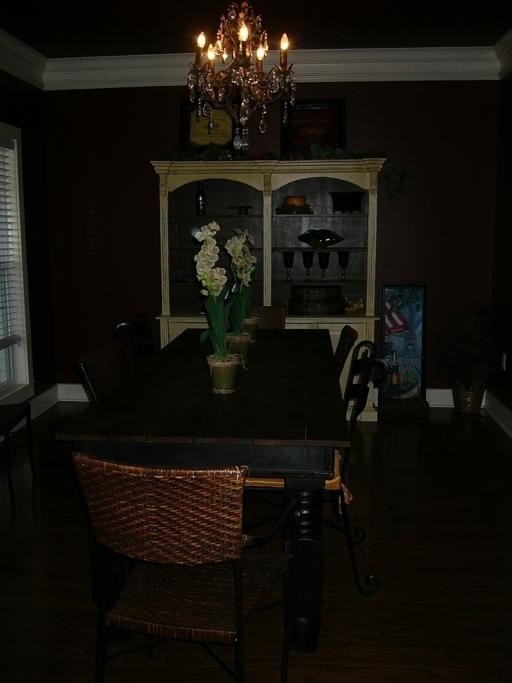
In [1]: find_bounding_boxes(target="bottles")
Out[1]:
[196,183,207,216]
[389,352,400,402]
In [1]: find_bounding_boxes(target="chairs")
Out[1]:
[73,303,395,420]
[74,449,328,681]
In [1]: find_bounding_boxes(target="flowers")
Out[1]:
[188,220,257,358]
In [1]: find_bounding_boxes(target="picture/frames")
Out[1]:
[279,98,348,159]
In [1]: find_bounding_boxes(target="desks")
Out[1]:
[55,327,354,656]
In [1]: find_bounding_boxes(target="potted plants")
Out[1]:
[438,302,496,416]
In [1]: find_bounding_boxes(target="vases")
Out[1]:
[206,330,252,396]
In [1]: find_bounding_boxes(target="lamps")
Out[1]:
[184,1,299,151]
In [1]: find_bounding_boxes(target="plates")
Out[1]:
[298,229,343,250]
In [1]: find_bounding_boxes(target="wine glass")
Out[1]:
[283,250,350,283]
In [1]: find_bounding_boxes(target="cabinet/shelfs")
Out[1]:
[148,157,389,422]
